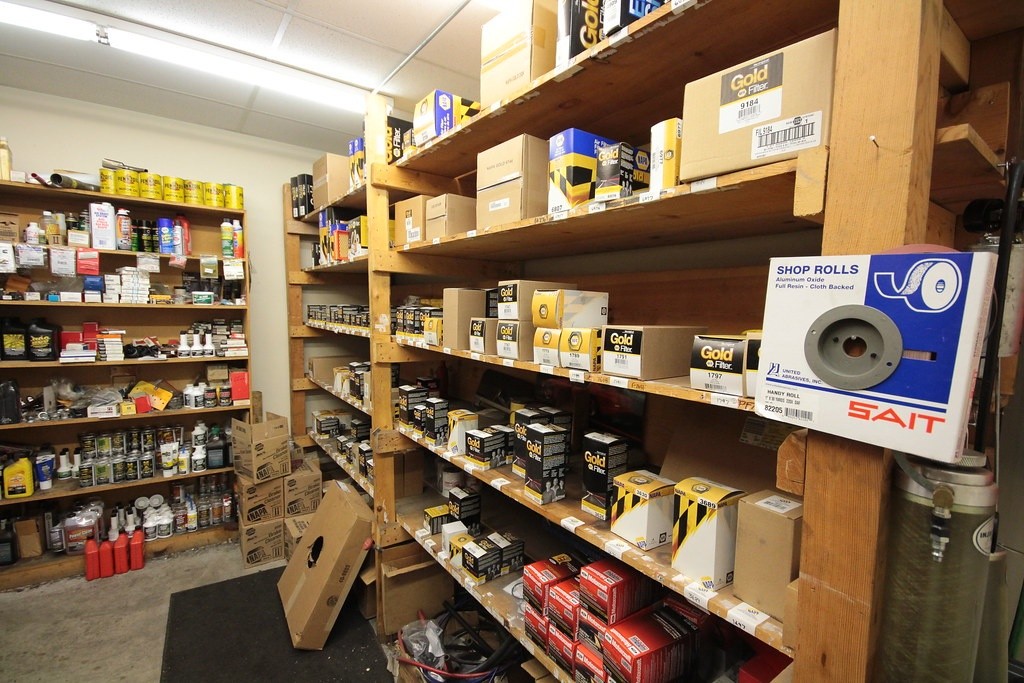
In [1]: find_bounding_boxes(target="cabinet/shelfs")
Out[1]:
[281,0,1024,683]
[0,179,252,592]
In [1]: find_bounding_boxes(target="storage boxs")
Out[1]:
[236,475,285,526]
[284,511,315,562]
[277,478,377,651]
[231,411,292,486]
[283,458,323,519]
[12,515,47,559]
[0,201,250,419]
[237,510,286,569]
[291,0,998,683]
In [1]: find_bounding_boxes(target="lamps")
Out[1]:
[0,0,394,116]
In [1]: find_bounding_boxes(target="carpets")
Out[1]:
[159,565,396,683]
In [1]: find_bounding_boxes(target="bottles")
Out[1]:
[183,382,208,410]
[116,207,132,251]
[0,442,56,500]
[26,318,61,361]
[220,217,234,259]
[57,447,82,480]
[172,210,192,256]
[0,378,20,425]
[0,316,28,360]
[26,209,91,246]
[183,492,198,532]
[177,329,215,359]
[0,494,145,581]
[0,136,13,181]
[233,219,243,258]
[176,419,208,476]
